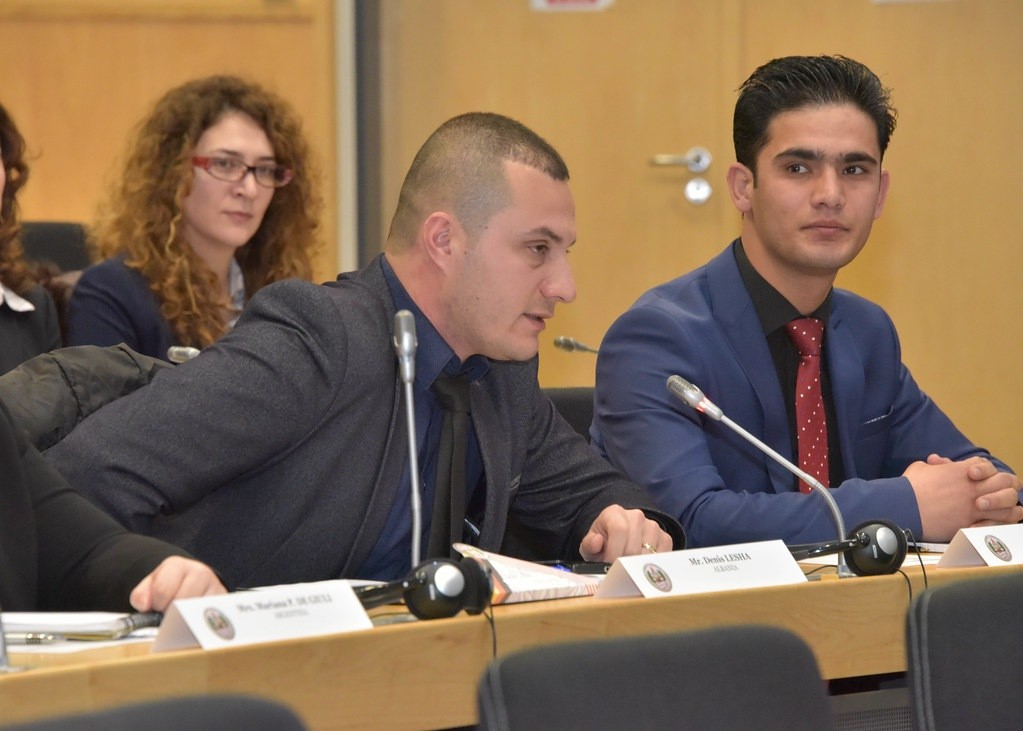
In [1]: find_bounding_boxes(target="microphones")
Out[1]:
[553,337,599,357]
[392,309,423,571]
[167,344,203,365]
[665,375,850,576]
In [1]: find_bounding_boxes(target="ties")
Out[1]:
[429,372,472,559]
[784,317,830,492]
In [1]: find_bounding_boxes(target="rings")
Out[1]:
[642,543,657,554]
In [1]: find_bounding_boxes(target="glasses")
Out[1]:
[193,157,296,188]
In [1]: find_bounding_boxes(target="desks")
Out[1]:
[0,548,1023,730]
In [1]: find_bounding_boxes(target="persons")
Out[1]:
[0,71,692,622]
[588,54,1023,560]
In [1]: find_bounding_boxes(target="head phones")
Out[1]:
[778,518,910,576]
[348,556,496,621]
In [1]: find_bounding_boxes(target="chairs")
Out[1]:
[20,567,1023,729]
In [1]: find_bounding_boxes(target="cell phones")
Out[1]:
[537,557,610,575]
